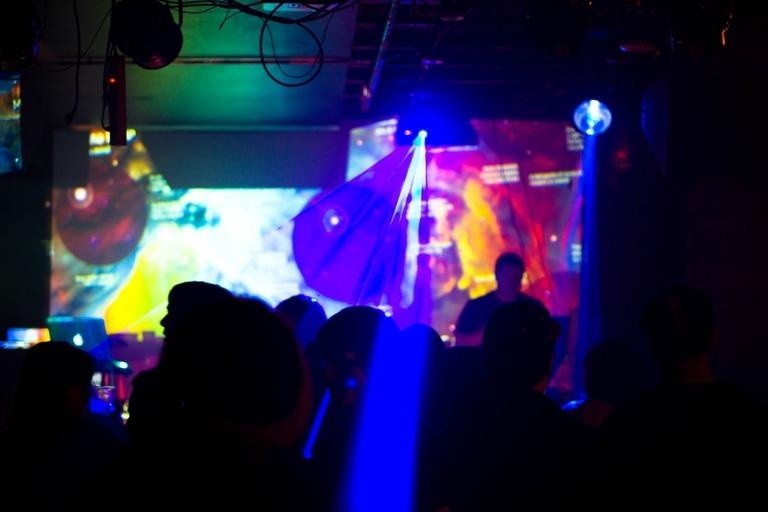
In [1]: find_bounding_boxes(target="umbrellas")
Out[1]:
[275,128,424,322]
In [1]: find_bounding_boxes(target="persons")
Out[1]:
[0,282,768,512]
[450,250,550,351]
